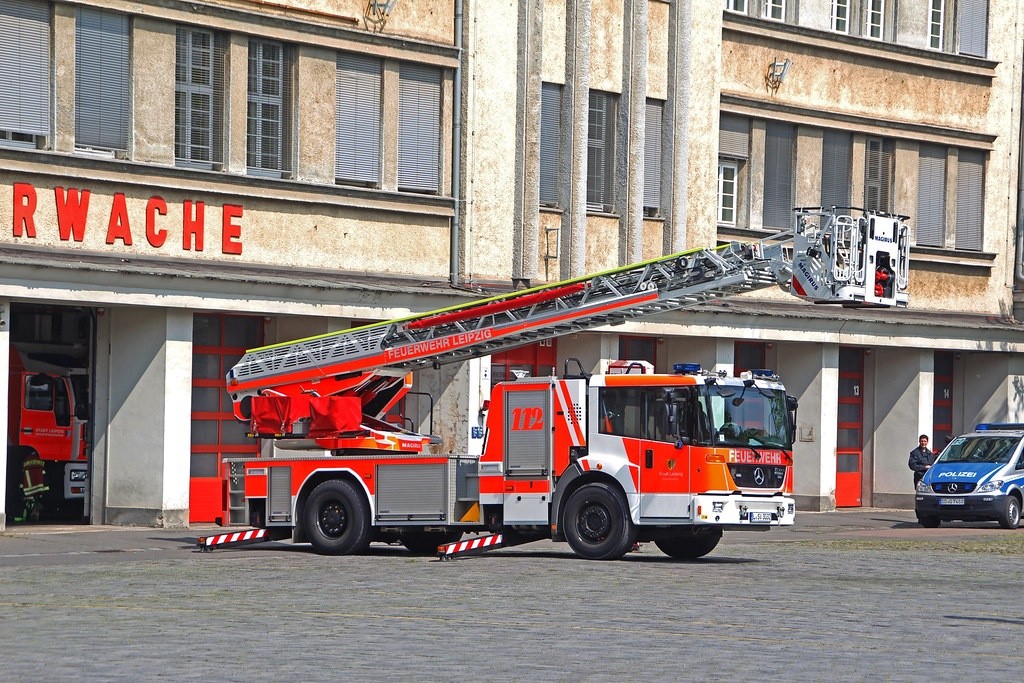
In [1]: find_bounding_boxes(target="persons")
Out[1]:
[908,435,936,525]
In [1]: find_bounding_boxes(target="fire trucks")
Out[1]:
[197,201,917,561]
[5,342,93,525]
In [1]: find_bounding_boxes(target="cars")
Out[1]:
[913,421,1024,530]
